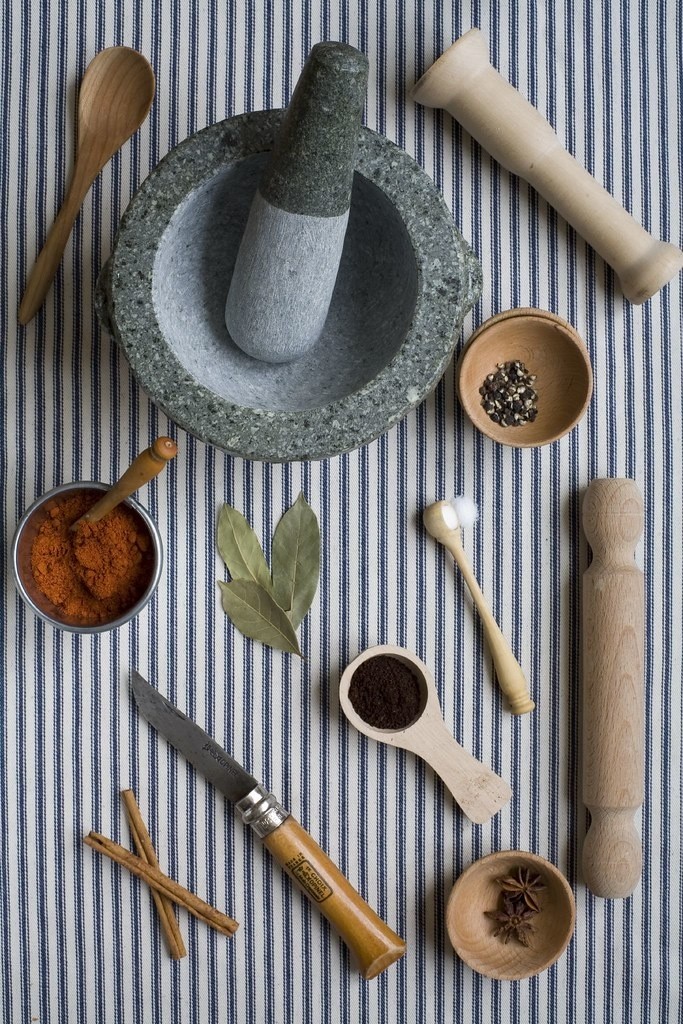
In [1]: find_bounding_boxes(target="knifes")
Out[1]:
[130,667,407,982]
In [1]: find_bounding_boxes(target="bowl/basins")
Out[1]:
[443,849,576,981]
[10,481,163,634]
[454,308,594,449]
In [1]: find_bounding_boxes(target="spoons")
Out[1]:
[18,48,156,325]
